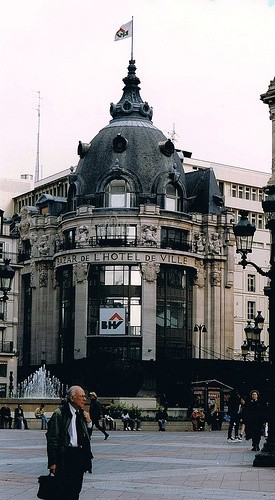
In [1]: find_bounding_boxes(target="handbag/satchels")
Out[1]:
[37,469,78,500]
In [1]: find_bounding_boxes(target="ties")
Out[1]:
[75,411,84,446]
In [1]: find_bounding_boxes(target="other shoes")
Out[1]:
[257,447,260,451]
[251,448,256,451]
[235,437,242,441]
[227,438,238,443]
[104,434,109,440]
[239,434,243,438]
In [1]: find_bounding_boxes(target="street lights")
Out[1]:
[234,184,275,467]
[241,311,267,389]
[193,324,208,358]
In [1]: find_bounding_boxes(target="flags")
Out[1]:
[114,20,133,41]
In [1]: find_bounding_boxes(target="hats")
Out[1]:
[90,392,97,399]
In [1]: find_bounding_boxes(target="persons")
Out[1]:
[85,392,109,441]
[206,408,217,431]
[45,386,94,500]
[198,409,205,431]
[0,404,13,429]
[155,407,168,431]
[129,409,141,431]
[213,408,223,430]
[14,404,29,429]
[35,404,48,429]
[246,390,266,452]
[191,410,202,431]
[100,404,116,431]
[226,390,243,442]
[236,397,253,440]
[121,409,135,431]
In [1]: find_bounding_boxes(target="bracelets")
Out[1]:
[86,419,92,423]
